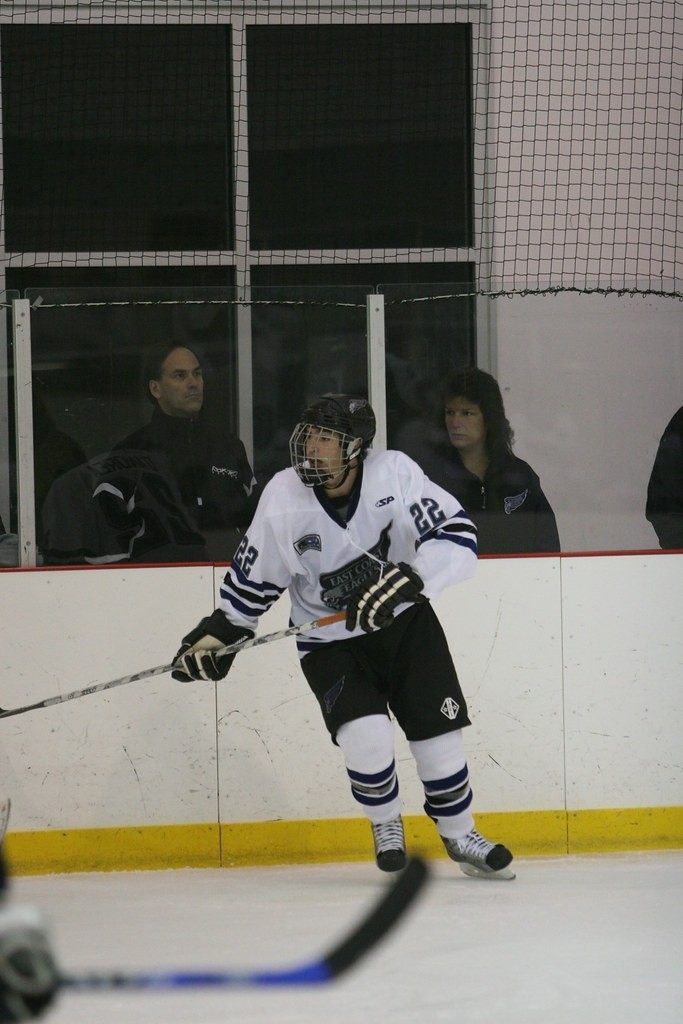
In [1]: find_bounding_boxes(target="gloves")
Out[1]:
[345,562,424,632]
[171,608,257,681]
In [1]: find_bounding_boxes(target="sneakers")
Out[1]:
[370,815,408,879]
[439,825,516,881]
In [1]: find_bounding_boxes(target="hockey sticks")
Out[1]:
[1,608,352,722]
[59,854,432,995]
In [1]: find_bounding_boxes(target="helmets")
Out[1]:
[288,391,376,488]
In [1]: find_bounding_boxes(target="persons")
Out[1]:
[97,340,264,563]
[418,370,560,554]
[172,395,516,881]
